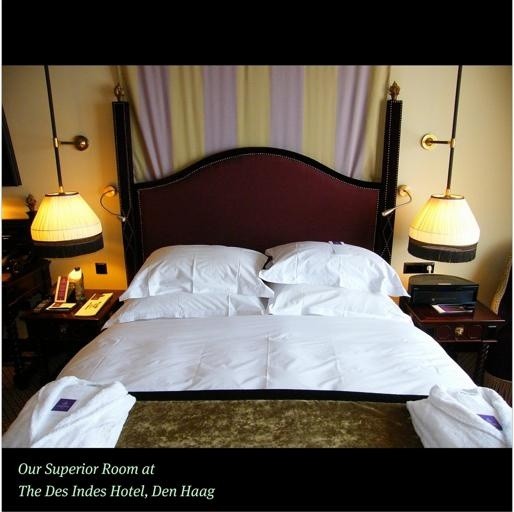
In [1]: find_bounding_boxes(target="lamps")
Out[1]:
[29,66,103,257]
[406,66,480,263]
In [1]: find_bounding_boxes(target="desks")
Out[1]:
[2,219,51,391]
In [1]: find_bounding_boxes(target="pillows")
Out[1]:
[100,294,265,330]
[259,238,412,297]
[265,282,407,316]
[119,244,275,301]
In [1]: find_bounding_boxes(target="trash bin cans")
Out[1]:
[483,367,512,406]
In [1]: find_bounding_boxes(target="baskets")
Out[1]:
[484,372,512,407]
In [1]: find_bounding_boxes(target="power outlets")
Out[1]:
[404,262,435,274]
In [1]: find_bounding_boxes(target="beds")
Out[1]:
[2,81,512,512]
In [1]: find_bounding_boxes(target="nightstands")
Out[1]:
[400,295,506,387]
[42,290,127,376]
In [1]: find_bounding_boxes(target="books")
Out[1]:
[44,301,77,313]
[430,303,474,314]
